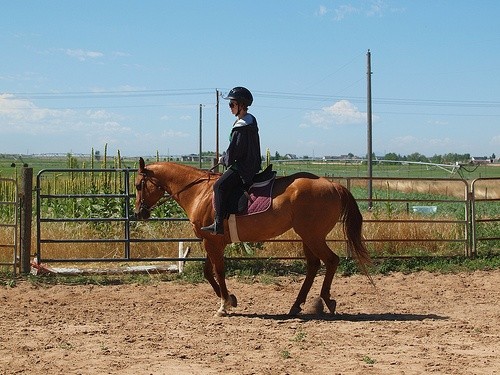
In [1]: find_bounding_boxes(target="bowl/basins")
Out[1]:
[413,206,438,213]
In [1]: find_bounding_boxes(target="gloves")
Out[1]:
[225,159,228,166]
[218,157,225,163]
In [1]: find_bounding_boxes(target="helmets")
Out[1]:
[224,87,253,106]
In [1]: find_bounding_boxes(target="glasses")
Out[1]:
[229,102,237,108]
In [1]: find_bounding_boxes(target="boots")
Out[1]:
[201,216,223,236]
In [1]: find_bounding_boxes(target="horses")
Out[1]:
[133,156,379,317]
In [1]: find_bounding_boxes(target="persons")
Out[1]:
[200,87,262,235]
